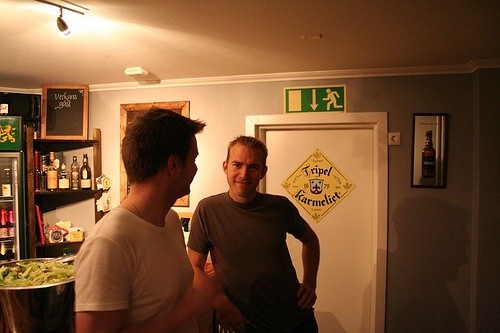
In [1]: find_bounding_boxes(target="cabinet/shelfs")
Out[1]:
[0,115,27,263]
[25,128,102,259]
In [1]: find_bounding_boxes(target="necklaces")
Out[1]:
[126,197,142,218]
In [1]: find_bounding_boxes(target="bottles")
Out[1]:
[0,241,15,259]
[2,169,12,197]
[46,152,57,189]
[71,156,79,190]
[422,131,436,177]
[58,162,70,189]
[80,154,92,190]
[0,209,15,237]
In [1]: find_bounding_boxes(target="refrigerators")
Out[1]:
[0,116,26,263]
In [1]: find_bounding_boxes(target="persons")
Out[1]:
[186,136,320,333]
[74,108,200,333]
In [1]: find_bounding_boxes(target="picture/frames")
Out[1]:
[410,112,452,188]
[120,101,190,207]
[41,83,89,141]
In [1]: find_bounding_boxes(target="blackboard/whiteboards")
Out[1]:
[41,83,89,140]
